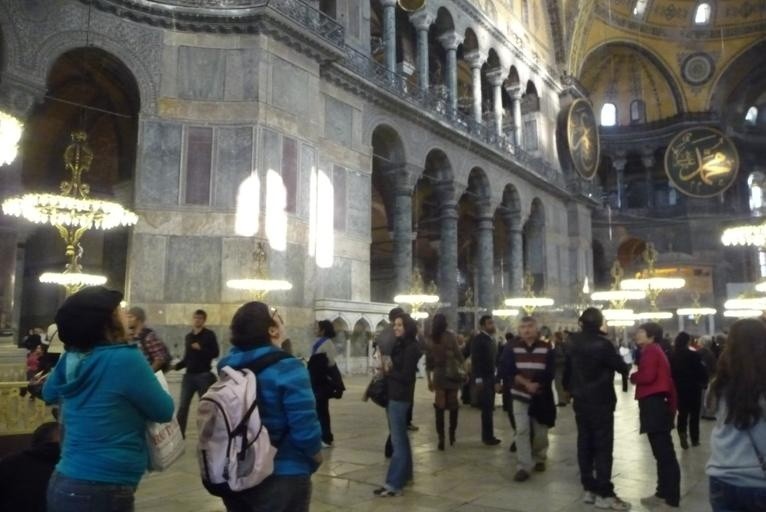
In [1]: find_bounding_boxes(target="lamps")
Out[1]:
[221,239,295,305]
[0,112,26,166]
[1,124,140,299]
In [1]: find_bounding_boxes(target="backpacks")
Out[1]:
[196,351,297,496]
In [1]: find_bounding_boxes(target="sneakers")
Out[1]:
[641,495,668,505]
[534,462,546,472]
[584,492,631,510]
[513,469,530,482]
[374,487,403,497]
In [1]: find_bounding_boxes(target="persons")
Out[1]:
[42,285,175,512]
[124,299,336,512]
[21,318,62,421]
[372,305,766,512]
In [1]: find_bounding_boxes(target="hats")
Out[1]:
[54,286,124,345]
[579,308,603,326]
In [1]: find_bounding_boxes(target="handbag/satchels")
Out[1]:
[444,359,469,382]
[370,380,387,408]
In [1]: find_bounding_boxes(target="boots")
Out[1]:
[449,407,458,444]
[434,403,445,450]
